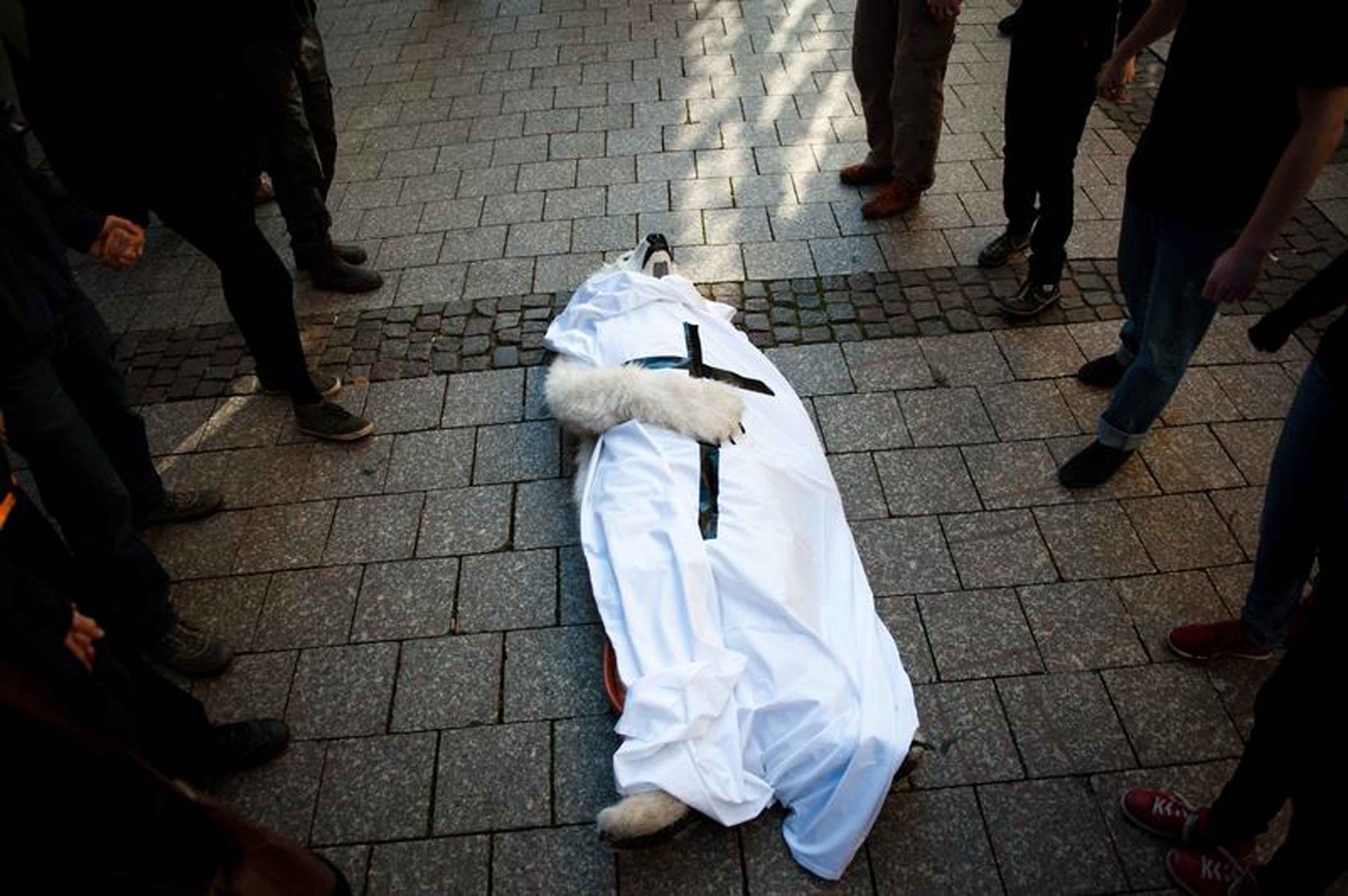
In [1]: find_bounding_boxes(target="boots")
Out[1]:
[296,232,383,293]
[289,238,368,270]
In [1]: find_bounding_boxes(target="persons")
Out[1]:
[0,0,382,896]
[837,0,1348,495]
[1118,253,1346,895]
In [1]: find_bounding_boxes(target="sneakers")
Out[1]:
[1166,847,1264,896]
[142,488,223,522]
[257,362,340,396]
[154,621,236,679]
[1121,784,1254,863]
[1286,591,1312,645]
[190,717,291,796]
[294,398,374,439]
[1059,437,1136,489]
[1168,619,1273,658]
[977,230,1033,268]
[1078,352,1125,388]
[838,161,892,185]
[998,279,1062,316]
[860,182,920,220]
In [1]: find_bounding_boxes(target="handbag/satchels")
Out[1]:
[206,826,336,896]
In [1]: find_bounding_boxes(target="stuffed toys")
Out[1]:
[544,228,929,847]
